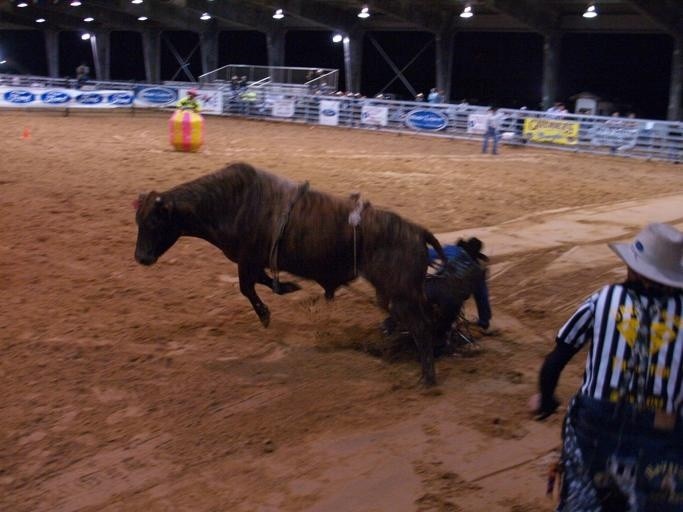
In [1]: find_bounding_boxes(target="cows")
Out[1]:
[134,162,466,388]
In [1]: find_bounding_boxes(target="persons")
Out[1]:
[605,110,622,125]
[480,102,502,156]
[550,103,563,110]
[414,92,422,102]
[230,75,240,113]
[77,60,90,90]
[240,75,249,88]
[556,105,571,115]
[176,88,204,114]
[625,113,638,128]
[364,236,492,355]
[527,222,683,512]
[433,87,447,105]
[426,88,436,102]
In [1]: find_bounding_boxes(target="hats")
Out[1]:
[455,235,490,264]
[605,223,683,290]
